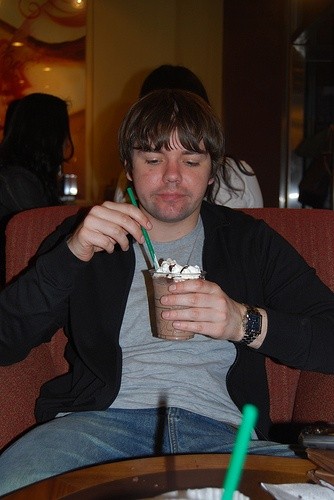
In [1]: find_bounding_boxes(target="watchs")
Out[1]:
[239,304,262,344]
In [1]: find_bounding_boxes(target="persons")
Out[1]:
[0,93,74,290]
[0,86,334,496]
[115,64,263,208]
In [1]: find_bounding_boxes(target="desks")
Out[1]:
[0,452,334,500]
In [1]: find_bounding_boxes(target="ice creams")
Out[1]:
[152,258,202,283]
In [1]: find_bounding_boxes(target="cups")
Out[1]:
[64,173,79,196]
[149,268,207,342]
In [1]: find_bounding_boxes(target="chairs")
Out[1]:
[0,203,334,448]
